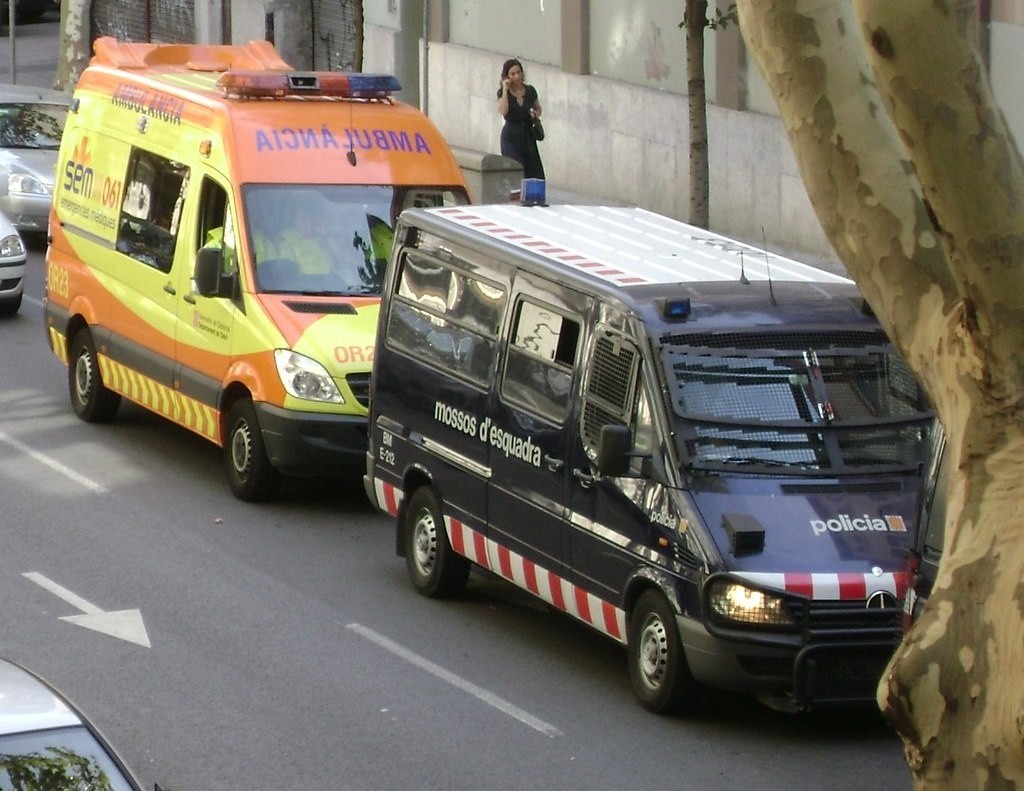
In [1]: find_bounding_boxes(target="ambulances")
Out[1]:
[41,37,477,504]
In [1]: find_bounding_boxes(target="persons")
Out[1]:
[199,195,403,275]
[497,60,546,180]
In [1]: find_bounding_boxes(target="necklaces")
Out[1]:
[511,88,522,94]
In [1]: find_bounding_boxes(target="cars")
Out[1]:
[0,655,167,791]
[0,209,28,317]
[1,82,72,233]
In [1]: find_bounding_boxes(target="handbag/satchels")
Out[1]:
[529,117,544,141]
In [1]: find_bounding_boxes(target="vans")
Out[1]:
[360,177,952,719]
